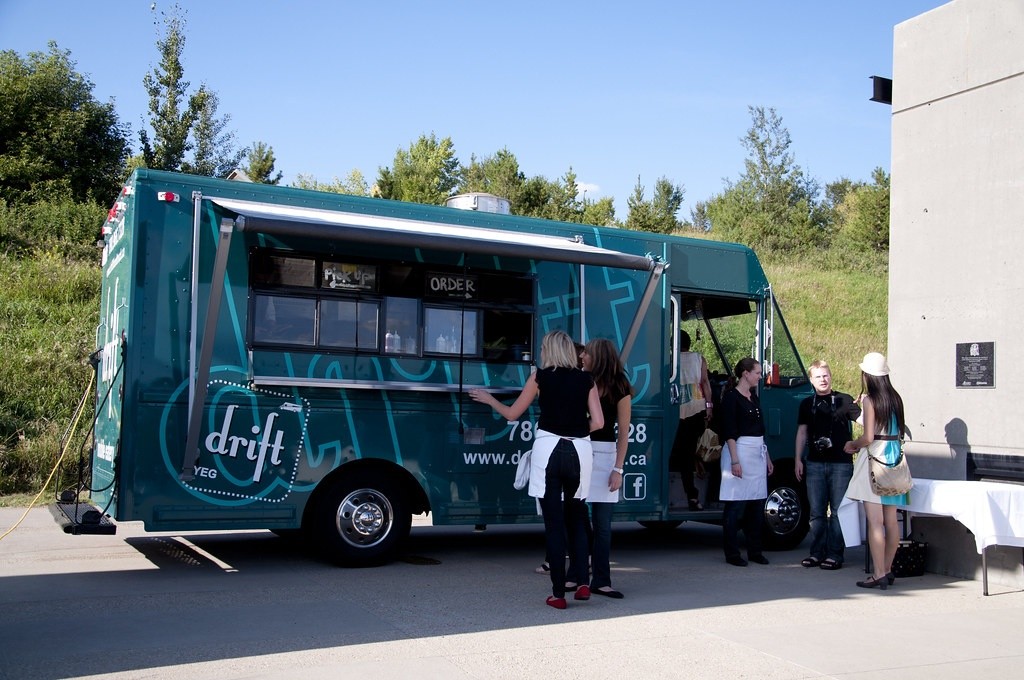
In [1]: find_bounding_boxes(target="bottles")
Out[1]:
[450,324,457,353]
[405,336,416,353]
[521,352,531,360]
[445,336,450,353]
[435,333,445,353]
[386,330,400,352]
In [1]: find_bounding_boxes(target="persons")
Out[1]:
[795,361,863,570]
[719,358,774,566]
[565,338,632,599]
[670,330,712,511]
[468,331,604,611]
[843,352,911,590]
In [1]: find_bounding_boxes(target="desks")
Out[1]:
[837,477,1024,596]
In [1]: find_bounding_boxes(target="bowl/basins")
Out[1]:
[483,348,506,359]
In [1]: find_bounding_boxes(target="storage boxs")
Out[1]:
[892,538,929,578]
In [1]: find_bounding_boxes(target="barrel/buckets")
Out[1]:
[513,345,531,360]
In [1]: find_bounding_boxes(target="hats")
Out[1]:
[859,353,890,376]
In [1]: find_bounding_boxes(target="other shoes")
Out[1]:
[564,584,578,592]
[726,557,748,567]
[574,586,590,600]
[590,585,624,599]
[546,596,567,609]
[748,556,769,564]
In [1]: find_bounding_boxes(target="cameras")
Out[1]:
[814,437,832,451]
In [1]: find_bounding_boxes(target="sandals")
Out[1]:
[801,556,822,567]
[820,558,842,570]
[535,564,551,574]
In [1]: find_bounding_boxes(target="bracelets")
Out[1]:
[706,402,713,408]
[732,462,738,465]
[613,467,623,474]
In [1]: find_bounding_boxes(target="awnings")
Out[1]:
[182,198,665,482]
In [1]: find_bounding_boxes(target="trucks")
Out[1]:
[46,165,823,568]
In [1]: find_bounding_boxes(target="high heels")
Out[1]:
[886,572,894,585]
[689,499,704,511]
[856,576,887,590]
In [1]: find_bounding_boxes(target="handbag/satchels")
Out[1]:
[868,453,913,496]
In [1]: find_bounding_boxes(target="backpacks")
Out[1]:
[695,420,722,479]
